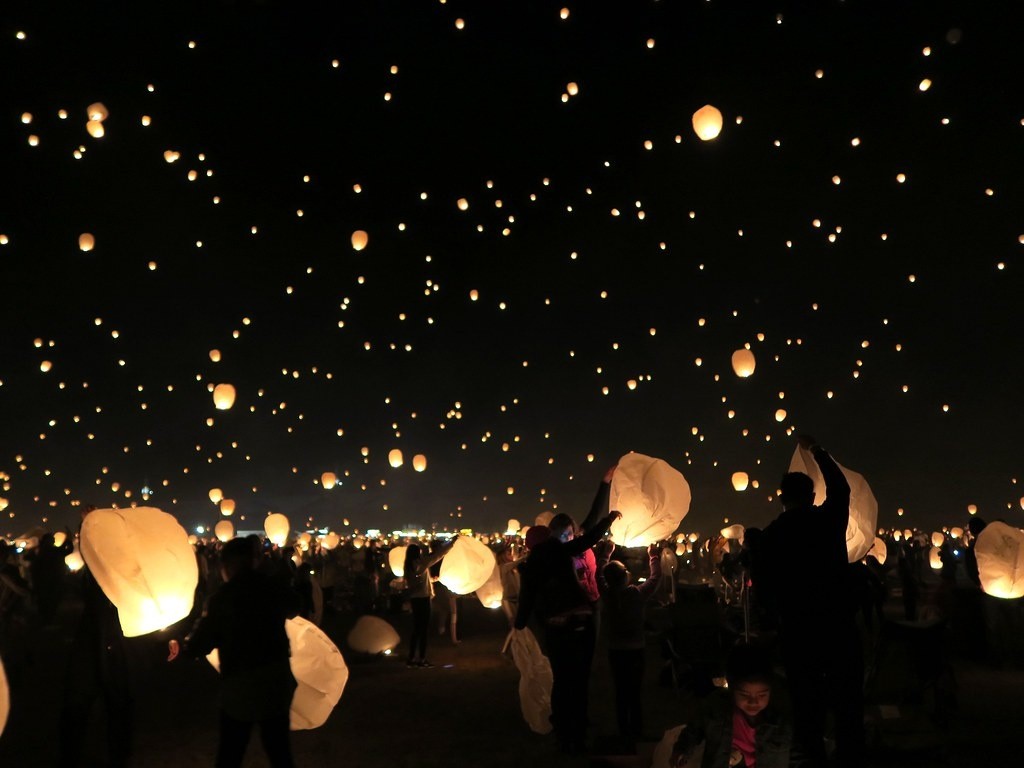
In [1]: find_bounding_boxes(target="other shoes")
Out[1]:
[406,660,418,668]
[418,659,435,669]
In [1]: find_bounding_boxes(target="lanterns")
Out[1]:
[0,0,1024,637]
[349,615,401,656]
[205,614,350,733]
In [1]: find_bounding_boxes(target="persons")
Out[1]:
[0,431,1024,768]
